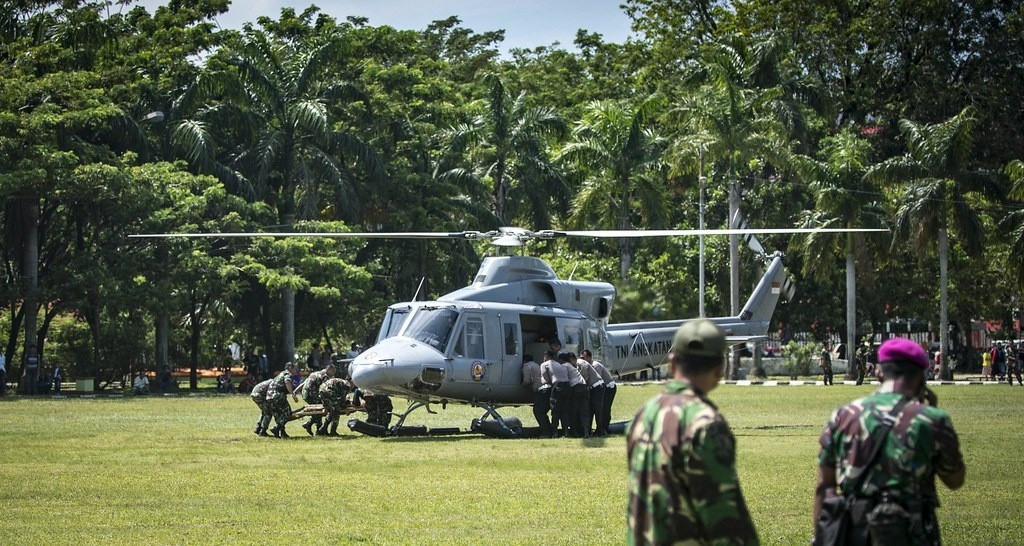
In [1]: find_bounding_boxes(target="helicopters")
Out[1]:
[128,225,890,438]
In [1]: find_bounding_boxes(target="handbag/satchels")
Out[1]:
[815,494,850,546]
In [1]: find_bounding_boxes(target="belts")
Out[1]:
[606,381,615,388]
[536,384,551,392]
[590,380,604,389]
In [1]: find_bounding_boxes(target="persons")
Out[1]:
[920,342,959,381]
[243,342,394,439]
[23,344,40,396]
[37,359,66,395]
[448,313,471,358]
[0,344,7,400]
[855,342,883,386]
[623,317,766,546]
[818,340,835,386]
[522,338,617,438]
[216,371,257,394]
[133,370,150,395]
[981,337,1024,387]
[813,337,966,546]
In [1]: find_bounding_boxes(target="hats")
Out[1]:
[674,319,726,356]
[877,338,928,369]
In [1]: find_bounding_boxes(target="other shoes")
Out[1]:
[281,430,289,437]
[318,426,328,435]
[259,429,270,437]
[254,428,261,435]
[270,426,281,439]
[302,422,314,436]
[329,429,339,436]
